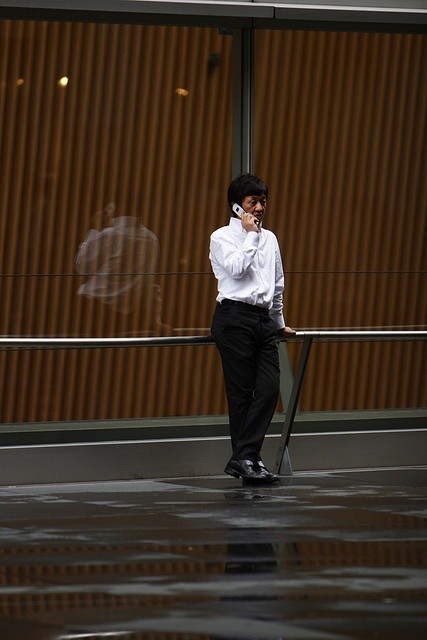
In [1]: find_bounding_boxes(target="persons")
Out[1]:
[71,192,174,339]
[210,173,296,483]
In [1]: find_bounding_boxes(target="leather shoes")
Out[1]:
[242,461,280,484]
[224,458,267,480]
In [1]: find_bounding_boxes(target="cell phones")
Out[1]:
[103,202,115,221]
[232,203,259,225]
